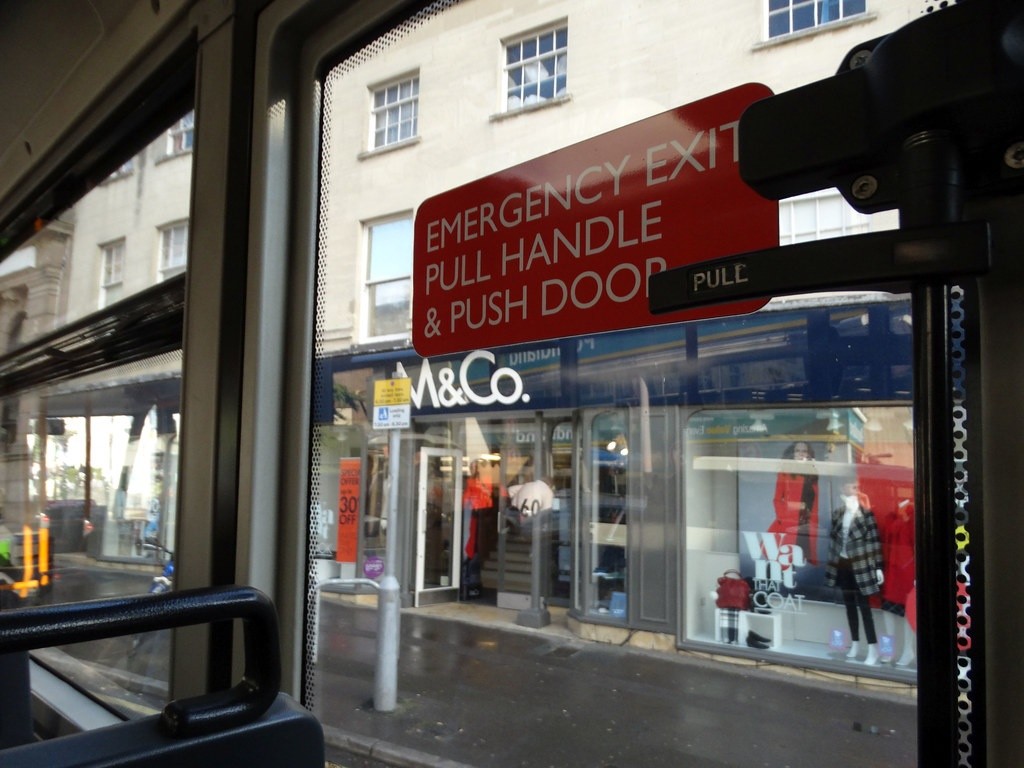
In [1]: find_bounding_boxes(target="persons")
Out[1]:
[881,500,970,665]
[824,484,884,664]
[759,441,819,595]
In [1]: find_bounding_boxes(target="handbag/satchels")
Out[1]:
[717,569,750,611]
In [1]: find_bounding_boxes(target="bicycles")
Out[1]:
[125,535,180,672]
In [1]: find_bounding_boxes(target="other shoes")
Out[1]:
[747,630,772,649]
